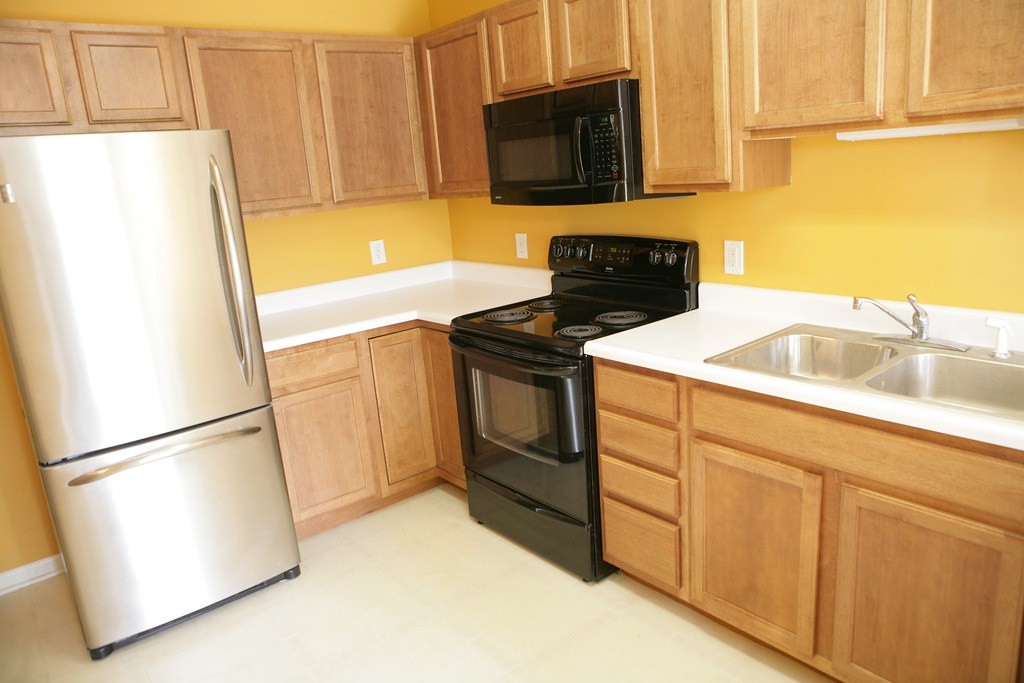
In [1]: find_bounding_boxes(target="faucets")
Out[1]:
[985,316,1014,360]
[853,292,972,352]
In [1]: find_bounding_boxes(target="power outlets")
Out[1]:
[515,233,528,259]
[724,240,745,275]
[369,240,386,265]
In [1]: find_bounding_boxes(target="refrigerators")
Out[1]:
[0,129,301,660]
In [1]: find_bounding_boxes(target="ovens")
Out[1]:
[448,333,619,583]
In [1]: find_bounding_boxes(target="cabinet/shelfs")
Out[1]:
[0,0,1024,212]
[265,321,468,537]
[589,359,1024,682]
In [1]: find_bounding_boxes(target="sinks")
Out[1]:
[865,353,1024,414]
[703,320,898,382]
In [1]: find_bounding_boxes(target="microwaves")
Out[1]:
[482,77,645,207]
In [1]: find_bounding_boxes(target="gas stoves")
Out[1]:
[449,234,700,361]
